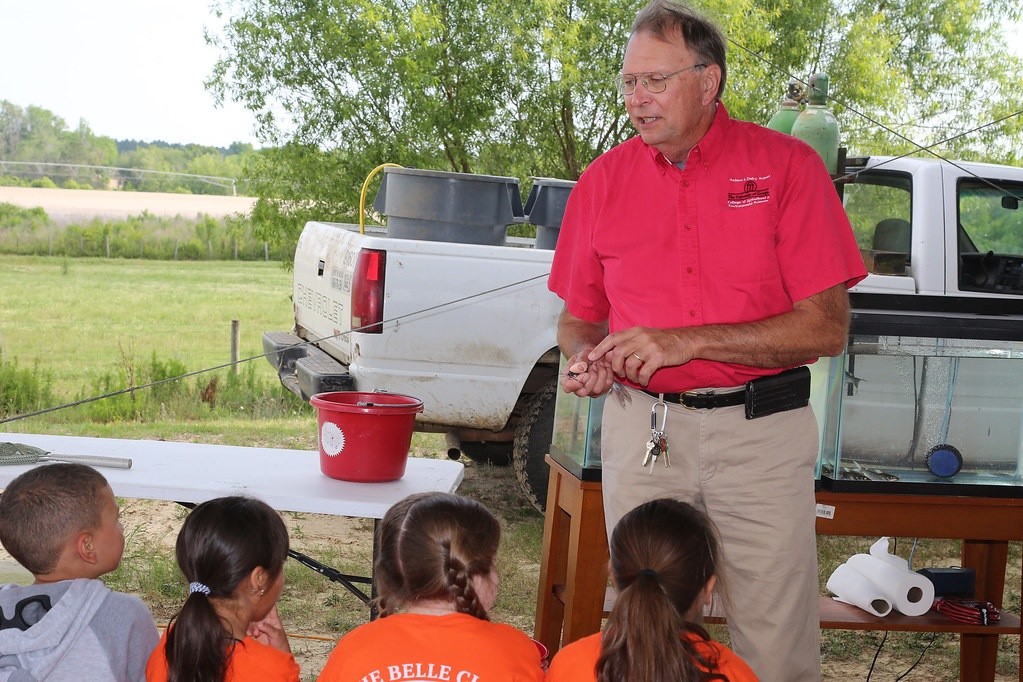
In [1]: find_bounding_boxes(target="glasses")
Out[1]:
[615,63,709,95]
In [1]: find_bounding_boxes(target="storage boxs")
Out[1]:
[821,292,1023,489]
[550,352,830,480]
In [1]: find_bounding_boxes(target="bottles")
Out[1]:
[766,81,801,137]
[791,73,838,194]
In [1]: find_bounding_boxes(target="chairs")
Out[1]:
[873,216,913,261]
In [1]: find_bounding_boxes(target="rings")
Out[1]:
[633,352,642,361]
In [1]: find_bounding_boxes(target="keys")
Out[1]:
[643,437,671,476]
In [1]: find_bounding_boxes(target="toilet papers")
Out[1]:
[846,536,935,617]
[825,563,893,618]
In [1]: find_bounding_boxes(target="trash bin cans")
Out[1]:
[373,166,524,246]
[523,179,577,250]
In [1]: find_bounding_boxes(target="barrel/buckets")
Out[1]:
[306,391,425,482]
[382,166,520,247]
[533,178,579,250]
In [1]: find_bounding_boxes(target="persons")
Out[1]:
[546,499,758,682]
[547,0,869,682]
[317,492,545,682]
[144,496,300,682]
[0,464,158,682]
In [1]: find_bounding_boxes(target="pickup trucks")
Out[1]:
[261,143,1023,520]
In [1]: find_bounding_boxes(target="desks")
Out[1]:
[533,454,1023,682]
[0,432,467,621]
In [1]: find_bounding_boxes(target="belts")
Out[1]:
[639,388,746,410]
